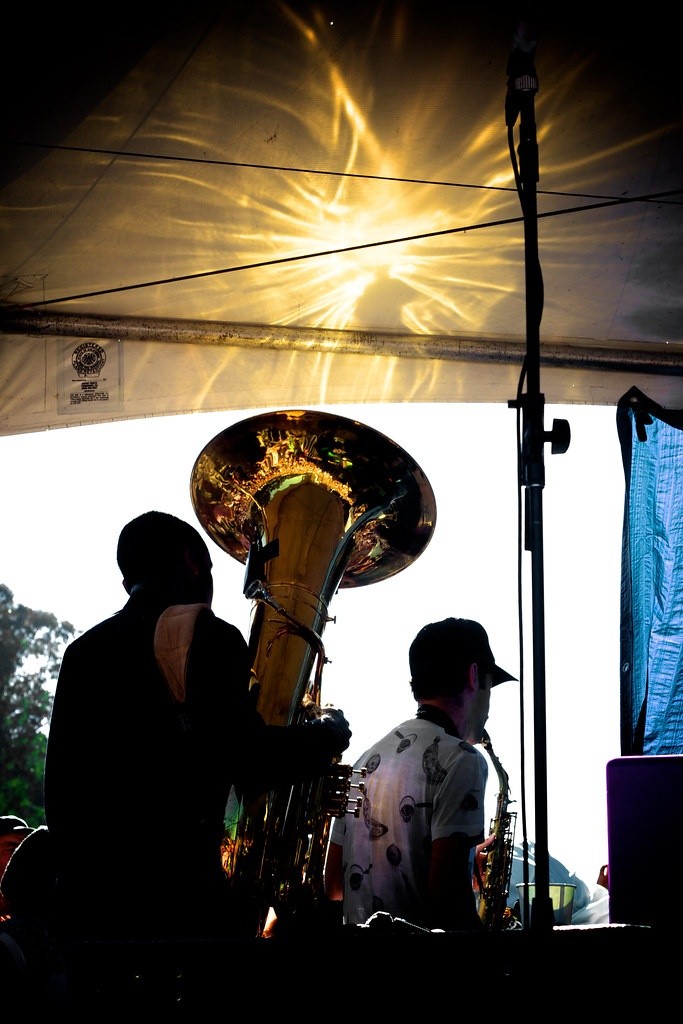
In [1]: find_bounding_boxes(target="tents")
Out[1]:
[1,0,681,438]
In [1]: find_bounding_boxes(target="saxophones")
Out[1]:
[479,730,521,933]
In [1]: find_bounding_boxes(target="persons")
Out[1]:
[323,617,518,972]
[0,813,37,906]
[41,510,353,1023]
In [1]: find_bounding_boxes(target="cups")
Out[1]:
[516,883,576,924]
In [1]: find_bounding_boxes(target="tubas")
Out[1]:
[191,408,435,912]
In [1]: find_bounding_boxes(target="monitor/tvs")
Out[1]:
[607,755,683,926]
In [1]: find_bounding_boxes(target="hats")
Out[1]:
[409,617,519,688]
